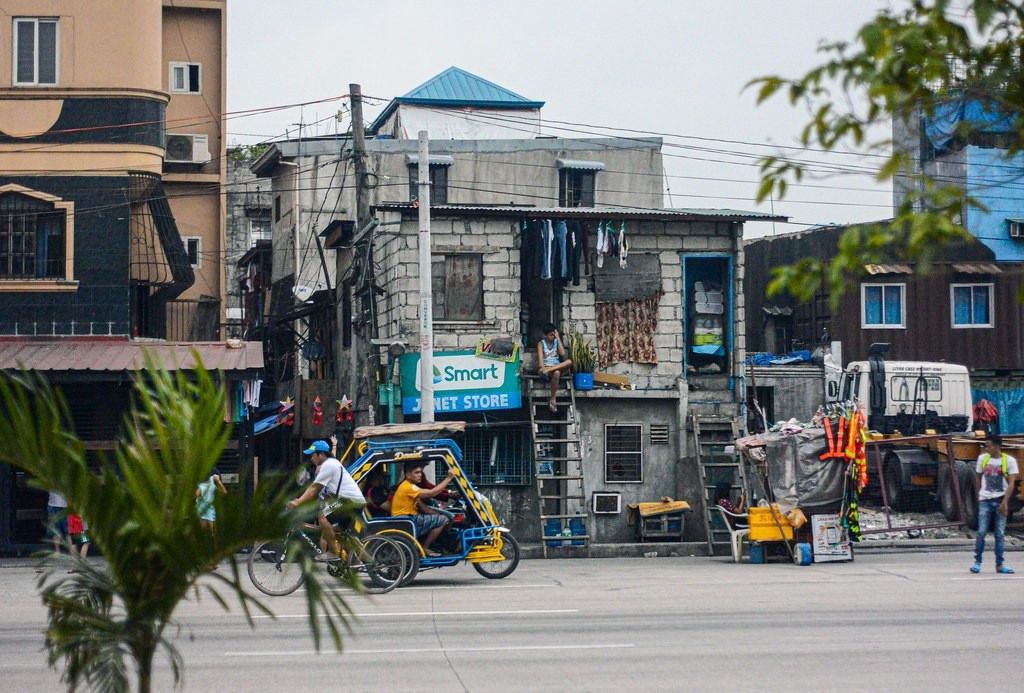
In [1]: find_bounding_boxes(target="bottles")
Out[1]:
[562,524,572,545]
[544,513,562,546]
[568,511,586,543]
[827,527,837,545]
[753,489,757,507]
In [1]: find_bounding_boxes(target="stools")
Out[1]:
[759,541,795,563]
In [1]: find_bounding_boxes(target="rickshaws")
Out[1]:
[326,421,521,588]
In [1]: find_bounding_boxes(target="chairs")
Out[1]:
[715,504,723,545]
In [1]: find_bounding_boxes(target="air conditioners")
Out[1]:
[164,133,211,164]
[592,493,621,514]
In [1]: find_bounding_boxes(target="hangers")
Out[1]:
[598,217,626,232]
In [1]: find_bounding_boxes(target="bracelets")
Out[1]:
[332,444,337,446]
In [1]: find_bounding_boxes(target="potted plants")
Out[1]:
[563,326,599,390]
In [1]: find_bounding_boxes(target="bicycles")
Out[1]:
[247,507,407,597]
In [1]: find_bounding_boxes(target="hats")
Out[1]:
[303,439,330,455]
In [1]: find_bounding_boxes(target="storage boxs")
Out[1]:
[688,282,724,345]
[748,506,793,540]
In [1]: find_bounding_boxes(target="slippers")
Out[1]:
[314,553,343,562]
[424,549,442,558]
[996,565,1014,573]
[971,564,982,573]
[541,371,549,380]
[549,400,557,413]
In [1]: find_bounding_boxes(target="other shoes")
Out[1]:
[67,567,76,574]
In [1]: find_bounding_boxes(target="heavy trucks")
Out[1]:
[836,342,1024,531]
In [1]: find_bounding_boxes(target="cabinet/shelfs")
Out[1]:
[636,506,685,542]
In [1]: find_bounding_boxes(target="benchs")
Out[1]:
[361,490,419,547]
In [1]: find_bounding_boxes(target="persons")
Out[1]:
[536,322,573,411]
[310,435,338,553]
[42,487,79,560]
[970,432,1020,574]
[64,501,96,573]
[369,458,460,557]
[712,483,745,552]
[286,441,367,572]
[193,467,229,568]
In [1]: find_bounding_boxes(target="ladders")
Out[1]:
[690,408,751,556]
[533,380,594,560]
